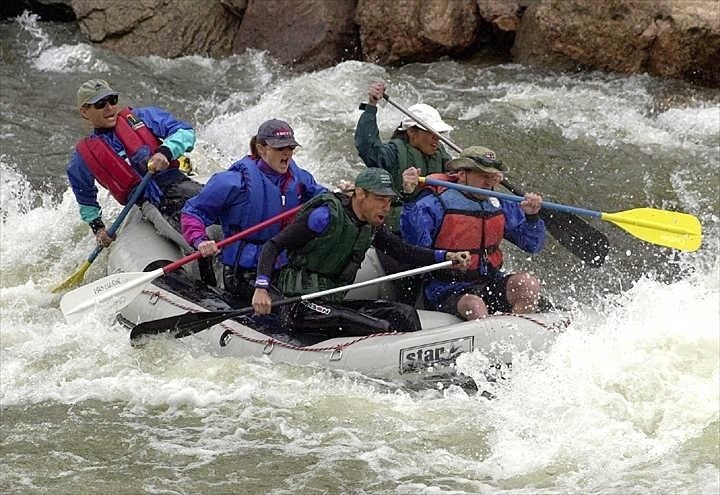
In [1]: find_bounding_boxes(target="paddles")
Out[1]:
[48,160,157,295]
[131,254,467,349]
[416,177,702,253]
[60,188,345,327]
[375,88,610,269]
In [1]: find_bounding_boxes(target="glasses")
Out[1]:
[94,94,118,109]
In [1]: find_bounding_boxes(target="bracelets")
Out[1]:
[254,275,271,289]
[433,249,447,262]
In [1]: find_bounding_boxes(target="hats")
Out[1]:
[77,79,120,109]
[398,104,454,132]
[258,119,303,150]
[445,145,510,173]
[355,167,396,196]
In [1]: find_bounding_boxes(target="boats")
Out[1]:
[108,221,565,389]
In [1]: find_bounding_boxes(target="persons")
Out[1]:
[66,78,206,255]
[178,119,355,330]
[353,81,505,240]
[401,144,547,320]
[252,167,472,341]
